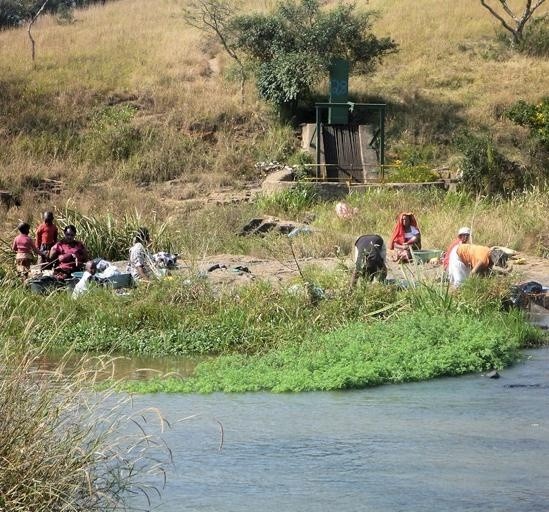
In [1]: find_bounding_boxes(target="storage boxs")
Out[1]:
[107,273,131,289]
[411,249,443,264]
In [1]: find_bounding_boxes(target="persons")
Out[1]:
[49,223,88,279]
[444,225,471,273]
[71,259,98,300]
[35,210,59,272]
[126,225,165,288]
[346,234,386,290]
[10,223,49,285]
[390,212,421,263]
[449,243,508,290]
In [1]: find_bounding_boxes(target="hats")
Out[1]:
[458,228,469,237]
